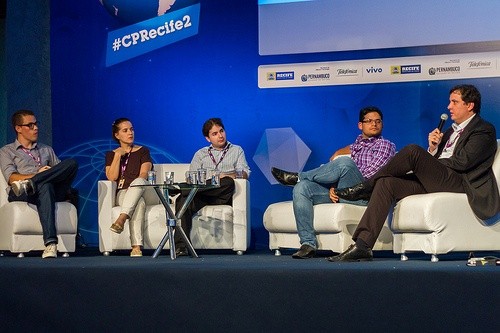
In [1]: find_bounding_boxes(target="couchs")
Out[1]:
[263,154,393,256]
[387,139,500,262]
[97,162,251,257]
[0,166,78,259]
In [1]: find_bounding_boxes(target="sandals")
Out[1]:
[130,250,142,257]
[110,223,124,234]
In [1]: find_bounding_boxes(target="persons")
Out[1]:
[327,84,500,263]
[105,117,162,257]
[0,109,78,258]
[164,118,252,256]
[271,105,397,260]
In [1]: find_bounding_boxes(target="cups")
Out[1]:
[211,169,219,185]
[197,168,208,185]
[185,171,189,184]
[147,170,156,185]
[189,171,198,184]
[234,164,244,179]
[165,171,174,184]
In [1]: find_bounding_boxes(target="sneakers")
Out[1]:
[41,243,57,258]
[12,179,30,196]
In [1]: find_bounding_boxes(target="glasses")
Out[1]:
[18,120,41,129]
[361,118,382,124]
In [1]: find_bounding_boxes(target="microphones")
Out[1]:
[432,114,448,145]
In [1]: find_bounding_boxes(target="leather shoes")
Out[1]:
[334,183,370,201]
[328,244,373,262]
[168,183,179,197]
[271,168,298,185]
[176,247,188,256]
[292,244,316,259]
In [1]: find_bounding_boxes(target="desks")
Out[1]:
[129,180,217,260]
